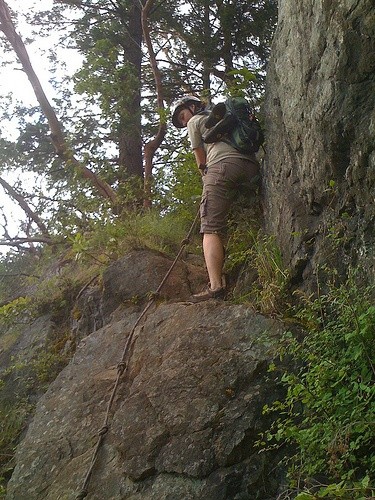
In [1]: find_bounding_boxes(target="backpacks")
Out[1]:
[195,96,267,155]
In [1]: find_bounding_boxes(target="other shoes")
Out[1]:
[202,115,237,143]
[205,102,226,129]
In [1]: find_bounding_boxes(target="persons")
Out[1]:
[171,95,262,303]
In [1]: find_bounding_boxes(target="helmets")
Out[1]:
[172,96,201,128]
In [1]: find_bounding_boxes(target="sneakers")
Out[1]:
[189,282,226,303]
